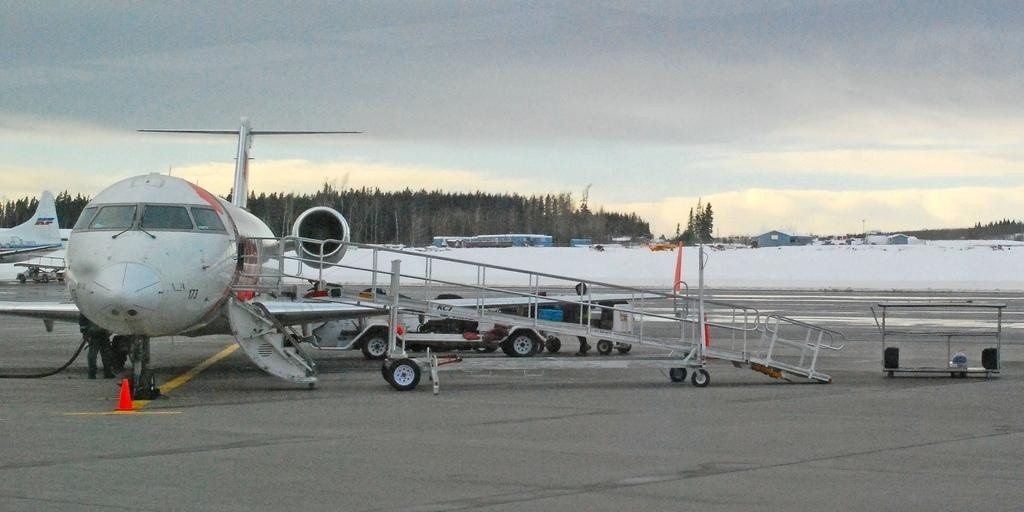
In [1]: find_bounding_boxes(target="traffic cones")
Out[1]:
[109,377,140,412]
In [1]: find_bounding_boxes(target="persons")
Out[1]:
[78,312,116,379]
[574,334,591,357]
